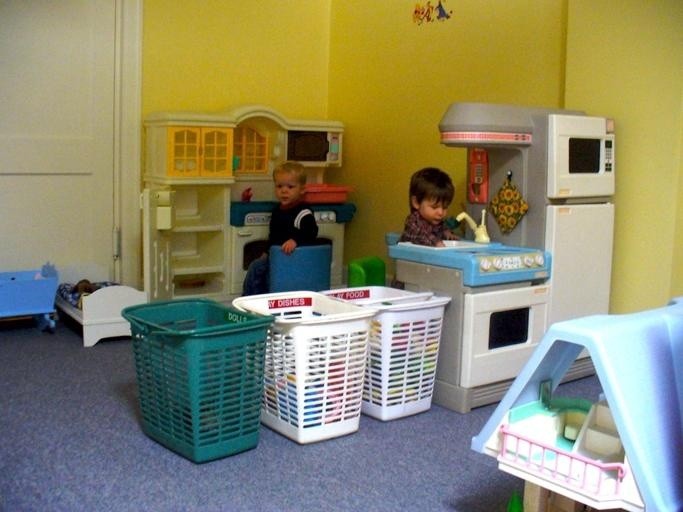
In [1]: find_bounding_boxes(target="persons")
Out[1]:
[388,168,465,289]
[240,161,319,296]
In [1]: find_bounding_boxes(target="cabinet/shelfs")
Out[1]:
[142,107,345,305]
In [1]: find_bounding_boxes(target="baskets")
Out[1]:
[123,300,272,462]
[321,285,451,422]
[232,291,371,446]
